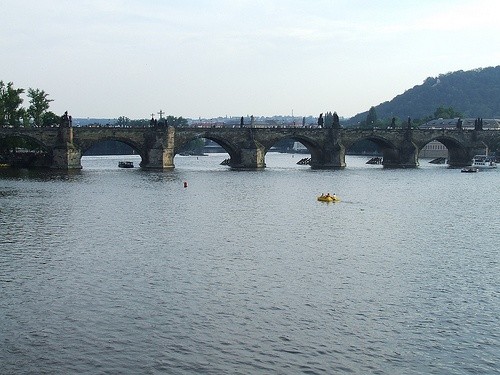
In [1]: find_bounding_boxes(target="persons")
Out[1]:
[320,192,336,198]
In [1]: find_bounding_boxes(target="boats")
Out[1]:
[473,156,497,169]
[317,194,338,202]
[461,166,477,173]
[118,161,133,168]
